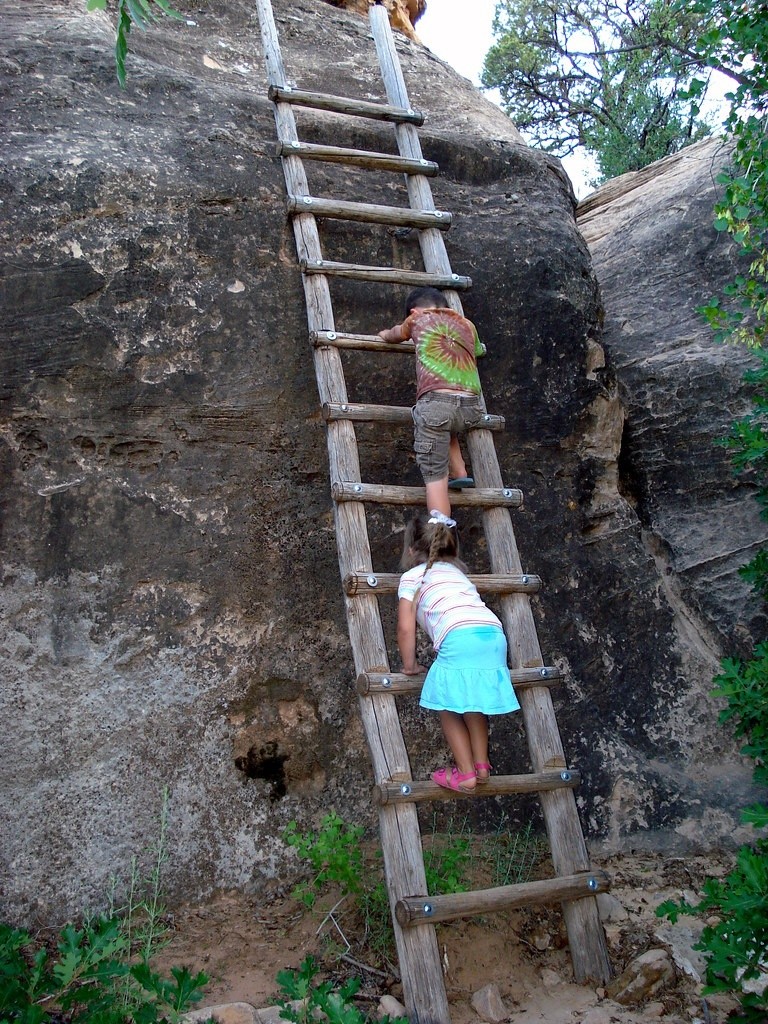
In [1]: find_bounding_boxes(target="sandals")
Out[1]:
[430,767,477,795]
[474,762,492,784]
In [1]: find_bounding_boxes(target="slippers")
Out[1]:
[448,478,473,489]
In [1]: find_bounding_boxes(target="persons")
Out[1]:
[396,509,521,794]
[377,288,484,520]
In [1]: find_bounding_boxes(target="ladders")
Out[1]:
[253,1,614,1023]
